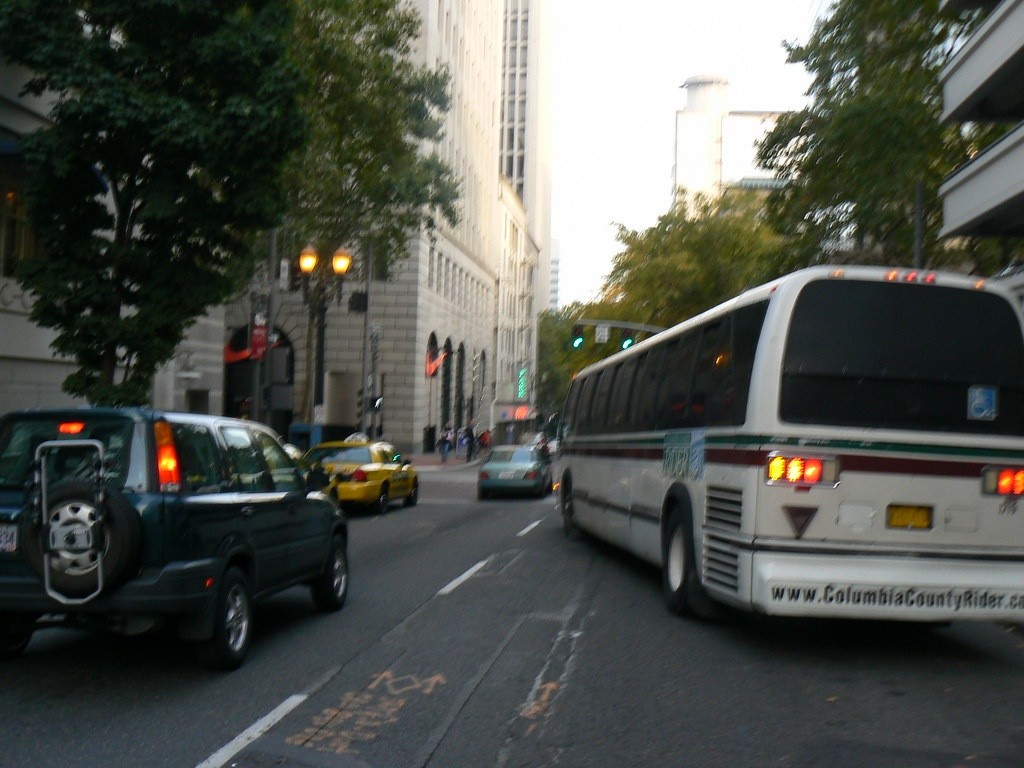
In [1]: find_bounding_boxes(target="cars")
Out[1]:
[304,433,419,515]
[476,443,552,499]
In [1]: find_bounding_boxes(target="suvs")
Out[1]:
[0,406,351,677]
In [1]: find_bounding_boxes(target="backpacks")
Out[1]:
[440,432,449,445]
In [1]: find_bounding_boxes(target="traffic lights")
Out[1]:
[571,325,584,350]
[619,330,634,351]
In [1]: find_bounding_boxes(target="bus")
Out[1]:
[554,261,1024,629]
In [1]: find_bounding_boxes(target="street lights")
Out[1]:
[296,246,351,425]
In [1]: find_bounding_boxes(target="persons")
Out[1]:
[440,423,451,462]
[466,421,474,463]
